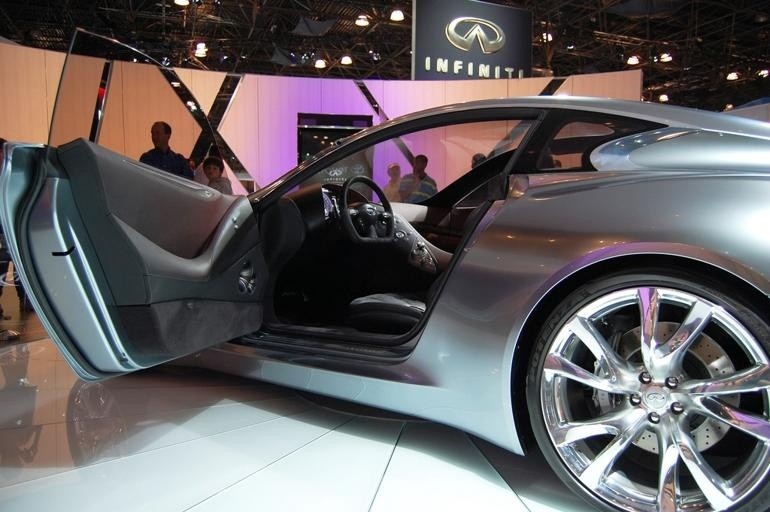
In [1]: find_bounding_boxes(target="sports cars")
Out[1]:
[0,26,769,512]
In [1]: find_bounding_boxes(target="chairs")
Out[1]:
[349,195,496,335]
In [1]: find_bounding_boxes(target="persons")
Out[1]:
[379,153,488,204]
[1,137,34,321]
[138,120,233,196]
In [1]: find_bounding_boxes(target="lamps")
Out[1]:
[355,11,369,27]
[315,57,327,68]
[340,53,352,65]
[389,6,405,21]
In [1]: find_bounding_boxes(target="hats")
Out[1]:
[387,162,400,169]
